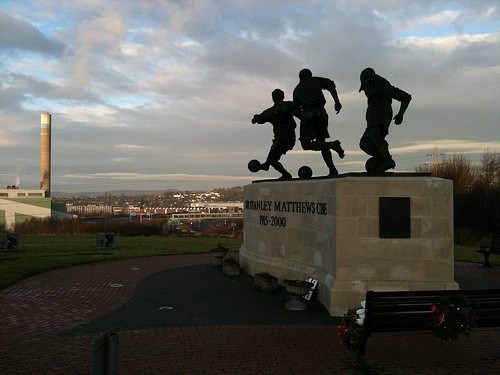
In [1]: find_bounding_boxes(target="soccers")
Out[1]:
[248,159,261,172]
[298,166,313,179]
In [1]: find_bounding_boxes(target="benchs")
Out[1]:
[354,289,500,375]
[477,228,500,268]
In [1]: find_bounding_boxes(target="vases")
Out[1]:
[284,279,313,310]
[222,255,239,275]
[255,273,277,292]
[211,242,229,266]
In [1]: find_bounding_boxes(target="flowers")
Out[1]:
[430,303,470,338]
[338,306,359,345]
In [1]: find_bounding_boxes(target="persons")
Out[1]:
[358,67,412,171]
[251,89,303,179]
[293,68,345,177]
